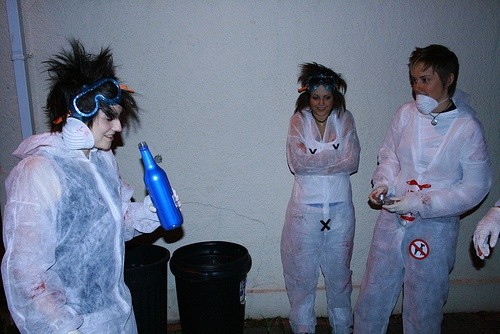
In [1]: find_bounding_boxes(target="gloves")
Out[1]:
[129,188,182,233]
[369,185,409,214]
[473,207,500,259]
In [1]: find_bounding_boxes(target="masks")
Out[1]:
[416,94,439,115]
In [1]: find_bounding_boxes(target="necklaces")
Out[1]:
[312,110,333,125]
[428,100,451,125]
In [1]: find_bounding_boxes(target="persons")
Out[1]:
[353,45,492,334]
[1,34,181,334]
[280,61,361,334]
[473,198,500,259]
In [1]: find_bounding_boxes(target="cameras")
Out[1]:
[376,193,398,204]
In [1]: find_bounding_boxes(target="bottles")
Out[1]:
[138,142,184,230]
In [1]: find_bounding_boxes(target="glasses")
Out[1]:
[73,79,121,121]
[307,76,336,91]
